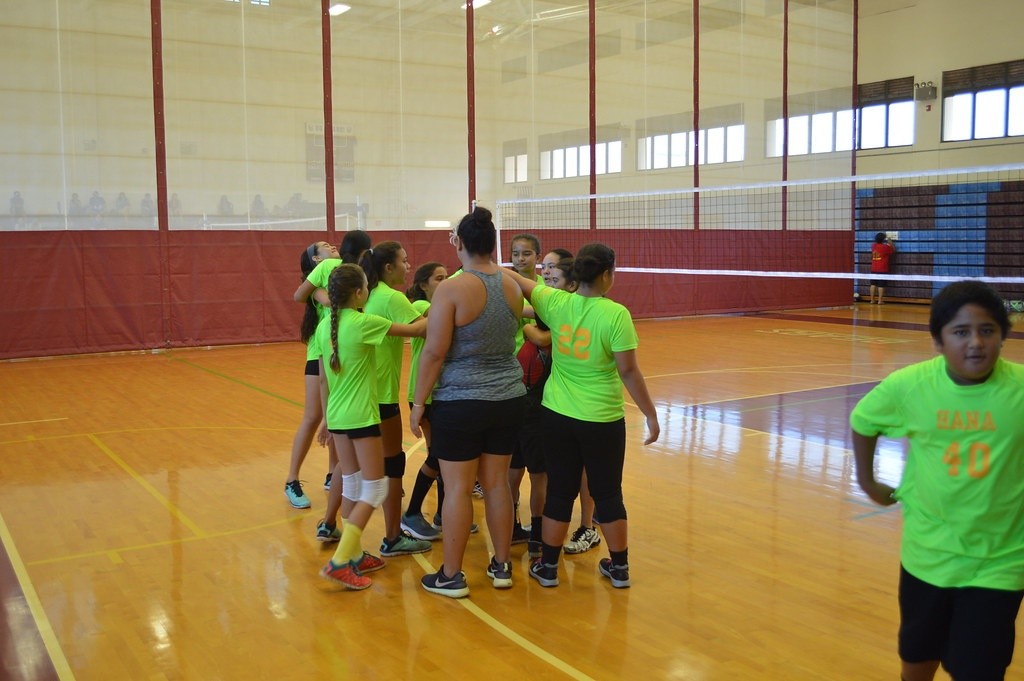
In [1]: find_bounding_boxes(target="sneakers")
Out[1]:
[530,557,558,586]
[512,522,532,544]
[431,513,478,532]
[563,525,601,553]
[599,558,630,587]
[472,482,487,498]
[380,529,432,556]
[528,538,543,557]
[487,555,512,587]
[422,565,469,598]
[324,473,333,490]
[401,514,440,541]
[319,560,372,590]
[286,479,311,508]
[401,487,405,497]
[350,550,386,574]
[316,518,341,541]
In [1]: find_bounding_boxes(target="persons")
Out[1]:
[850,280,1024,681]
[286,207,661,598]
[869,233,895,305]
